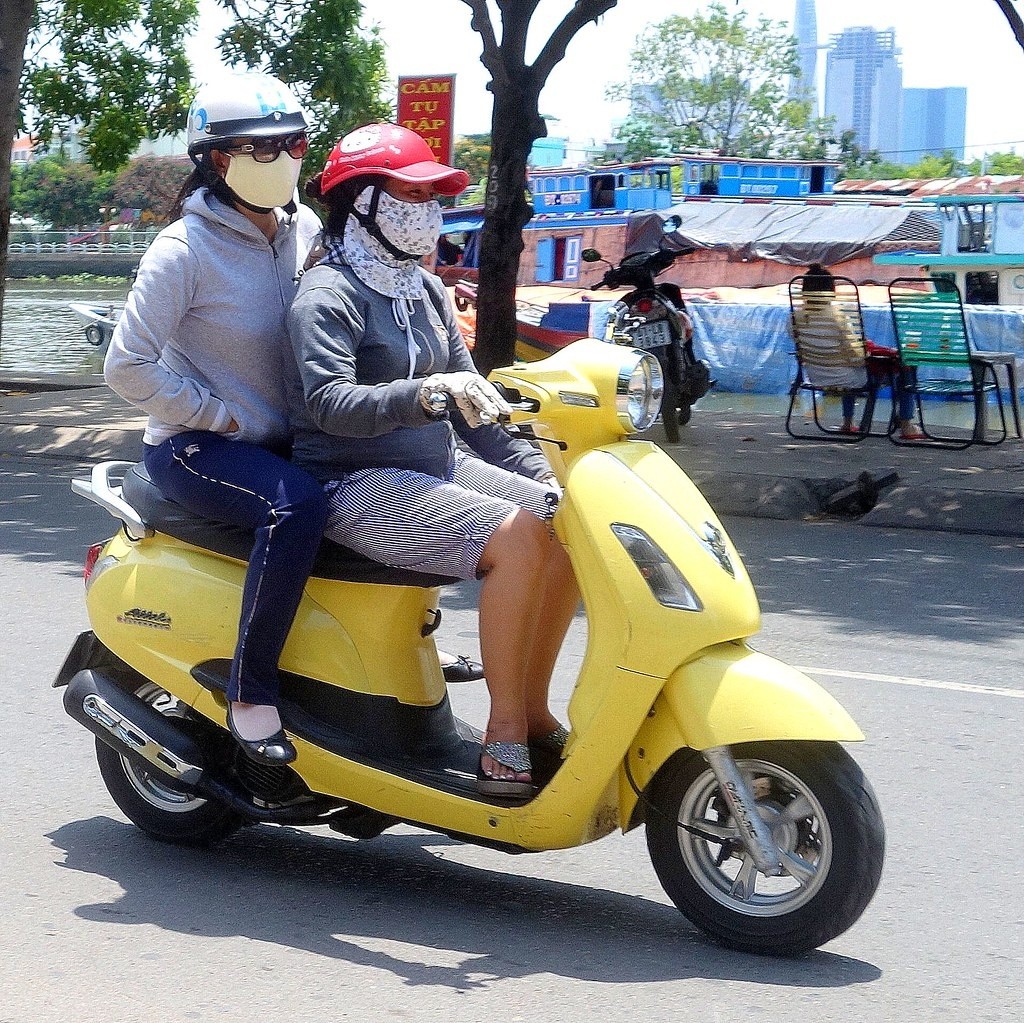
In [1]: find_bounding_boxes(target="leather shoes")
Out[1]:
[225,698,297,766]
[440,655,485,682]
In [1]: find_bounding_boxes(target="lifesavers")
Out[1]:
[85,325,104,346]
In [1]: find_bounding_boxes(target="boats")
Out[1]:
[873,193,1023,306]
[417,153,1024,405]
[68,301,127,353]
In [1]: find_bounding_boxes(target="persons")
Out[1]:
[104,73,487,767]
[787,265,927,437]
[282,124,581,797]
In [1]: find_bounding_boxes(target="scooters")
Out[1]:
[49,301,885,957]
[581,216,719,444]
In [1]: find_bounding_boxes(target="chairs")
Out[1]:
[887,278,1023,451]
[785,274,917,443]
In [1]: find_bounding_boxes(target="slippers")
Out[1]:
[841,425,859,432]
[530,724,572,755]
[476,744,533,800]
[900,429,929,440]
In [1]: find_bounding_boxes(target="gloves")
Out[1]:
[418,371,514,428]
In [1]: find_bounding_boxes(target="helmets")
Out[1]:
[323,123,470,195]
[187,70,308,155]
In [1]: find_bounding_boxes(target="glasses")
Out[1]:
[216,130,310,164]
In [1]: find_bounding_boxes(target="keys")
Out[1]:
[543,492,561,506]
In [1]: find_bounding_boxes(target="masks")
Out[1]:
[217,150,304,208]
